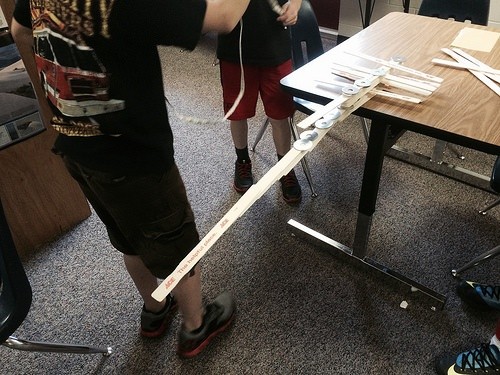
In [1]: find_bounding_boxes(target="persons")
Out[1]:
[442,279,500,375]
[218,0,304,204]
[11,0,249,358]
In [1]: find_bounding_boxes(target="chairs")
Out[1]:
[0,204,113,357]
[250,0,369,198]
[416,0,490,26]
[451,153,500,278]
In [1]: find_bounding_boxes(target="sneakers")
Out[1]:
[447,334,500,375]
[460,281,500,315]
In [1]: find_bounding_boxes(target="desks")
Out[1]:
[278,11,500,304]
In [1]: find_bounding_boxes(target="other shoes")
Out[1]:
[178,292,237,358]
[141,291,177,338]
[233,159,253,193]
[281,168,302,203]
